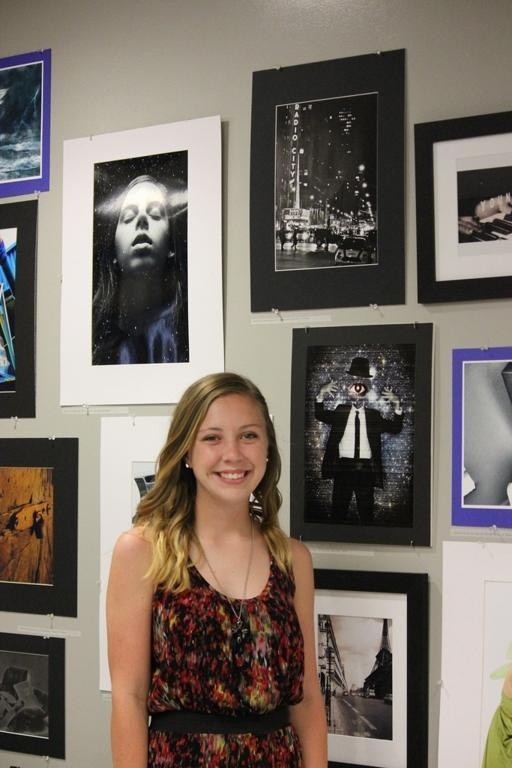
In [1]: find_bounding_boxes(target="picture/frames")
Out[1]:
[60,113,225,412]
[313,566,429,768]
[437,537,512,767]
[287,319,435,548]
[0,630,66,762]
[0,47,53,198]
[0,437,81,618]
[97,413,180,696]
[250,45,407,314]
[0,199,39,421]
[412,111,512,303]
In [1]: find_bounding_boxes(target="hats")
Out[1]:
[345,357,373,379]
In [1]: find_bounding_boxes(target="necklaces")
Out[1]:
[203,517,255,644]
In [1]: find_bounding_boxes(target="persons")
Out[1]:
[103,371,329,768]
[92,174,189,366]
[313,377,404,525]
